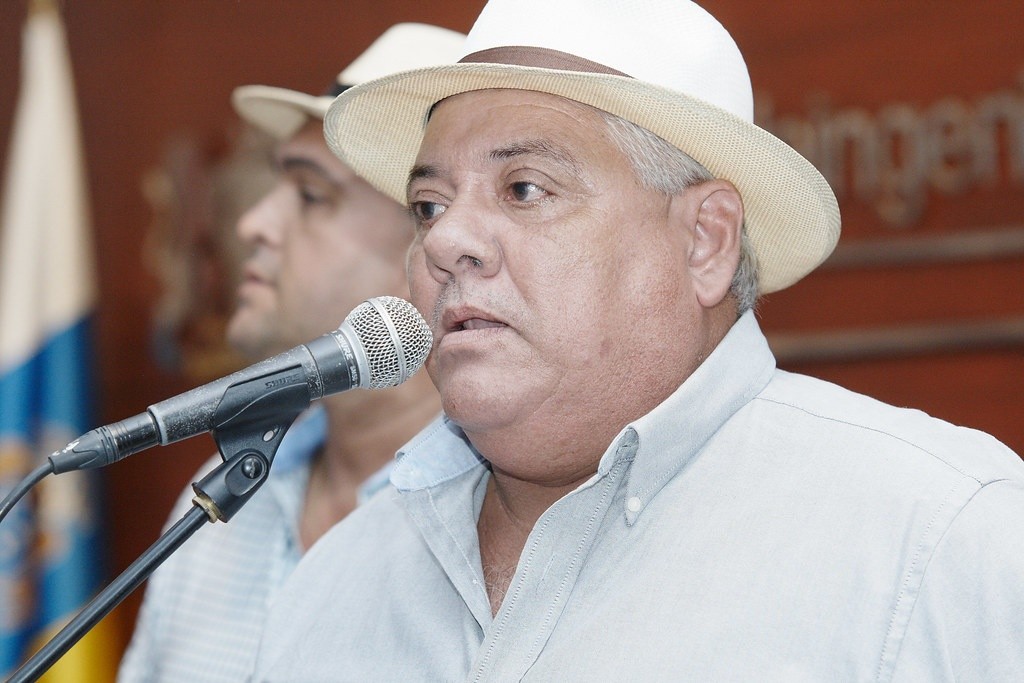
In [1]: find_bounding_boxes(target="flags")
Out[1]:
[0,0,119,683]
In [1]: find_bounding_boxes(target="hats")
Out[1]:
[230,22,468,143]
[322,0,842,297]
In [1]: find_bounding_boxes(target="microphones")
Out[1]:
[48,296,432,476]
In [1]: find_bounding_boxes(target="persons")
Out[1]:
[254,1,1024,683]
[117,23,466,683]
